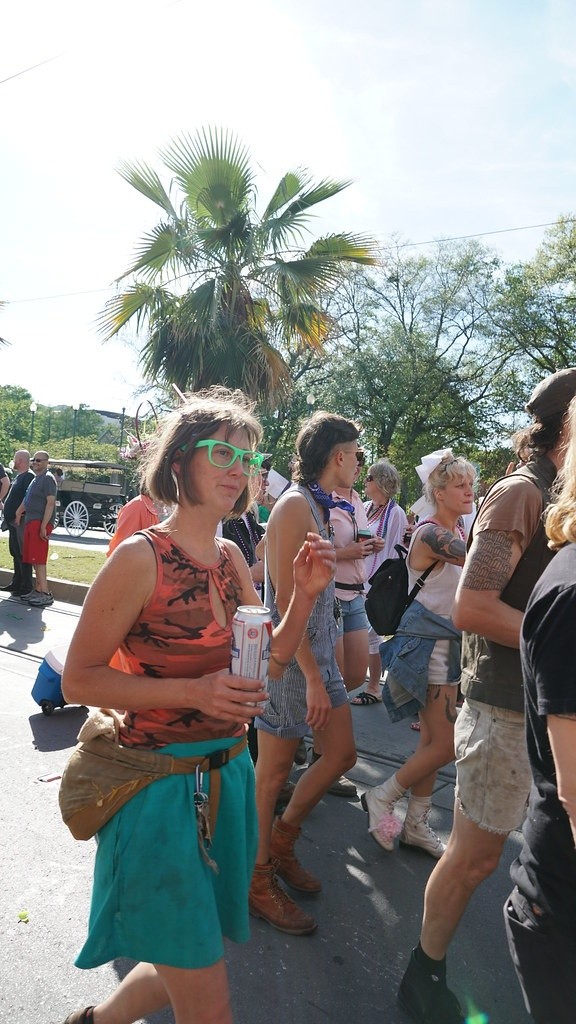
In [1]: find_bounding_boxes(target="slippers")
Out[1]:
[350,690,382,705]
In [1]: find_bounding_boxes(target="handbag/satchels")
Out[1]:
[60,715,250,842]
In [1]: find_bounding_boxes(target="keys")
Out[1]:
[195,793,221,875]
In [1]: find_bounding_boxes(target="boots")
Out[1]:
[399,795,448,858]
[247,858,316,935]
[396,945,463,1024]
[270,816,321,893]
[361,774,409,852]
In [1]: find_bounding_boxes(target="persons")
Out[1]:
[60,390,336,1023]
[0,450,35,597]
[360,447,513,859]
[500,393,576,1024]
[16,451,58,607]
[105,465,161,558]
[247,413,385,936]
[0,464,10,503]
[55,469,63,483]
[350,461,407,705]
[223,460,264,594]
[395,363,576,1023]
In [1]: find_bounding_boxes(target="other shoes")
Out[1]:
[309,747,358,797]
[0,581,19,591]
[293,737,307,765]
[12,588,32,596]
[410,721,420,729]
[63,1004,91,1024]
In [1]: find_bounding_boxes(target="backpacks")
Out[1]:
[364,520,441,636]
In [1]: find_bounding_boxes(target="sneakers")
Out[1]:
[21,590,39,601]
[29,591,54,606]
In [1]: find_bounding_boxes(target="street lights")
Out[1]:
[120,403,127,449]
[28,401,37,452]
[71,402,80,459]
[306,393,316,416]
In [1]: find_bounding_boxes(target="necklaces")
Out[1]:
[363,498,396,580]
[231,516,258,568]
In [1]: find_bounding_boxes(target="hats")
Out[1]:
[527,367,576,417]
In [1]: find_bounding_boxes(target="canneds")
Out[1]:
[357,529,372,556]
[229,605,273,708]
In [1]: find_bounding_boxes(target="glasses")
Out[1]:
[357,457,365,467]
[259,471,269,479]
[336,449,364,461]
[32,458,47,463]
[365,475,375,482]
[179,439,264,476]
